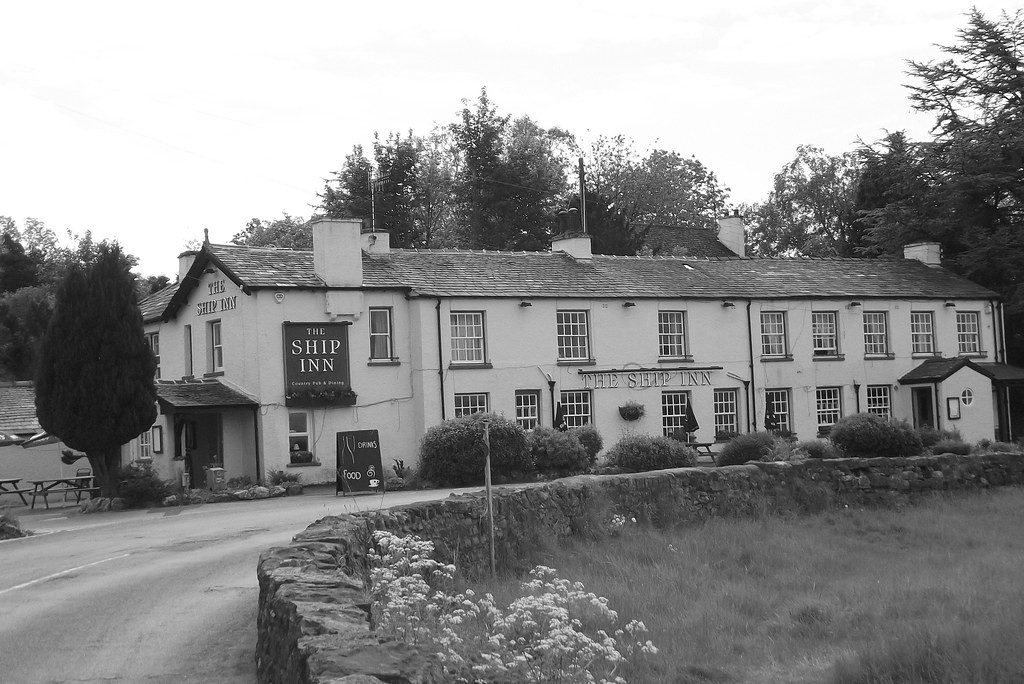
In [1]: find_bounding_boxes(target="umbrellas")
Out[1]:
[764,394,777,435]
[0,430,25,447]
[683,397,699,443]
[555,402,567,432]
[21,430,63,478]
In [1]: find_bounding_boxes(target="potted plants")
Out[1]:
[617,399,649,422]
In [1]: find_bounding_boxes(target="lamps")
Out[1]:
[622,302,636,307]
[848,302,862,307]
[520,302,533,308]
[721,303,735,307]
[943,303,956,308]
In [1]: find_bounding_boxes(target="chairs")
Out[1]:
[64,468,93,504]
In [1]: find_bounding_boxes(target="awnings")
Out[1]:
[978,362,1024,387]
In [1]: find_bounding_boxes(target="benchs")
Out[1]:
[695,451,721,457]
[0,488,31,496]
[28,486,102,496]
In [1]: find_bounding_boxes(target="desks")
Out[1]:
[26,476,96,510]
[0,478,29,507]
[685,442,718,468]
